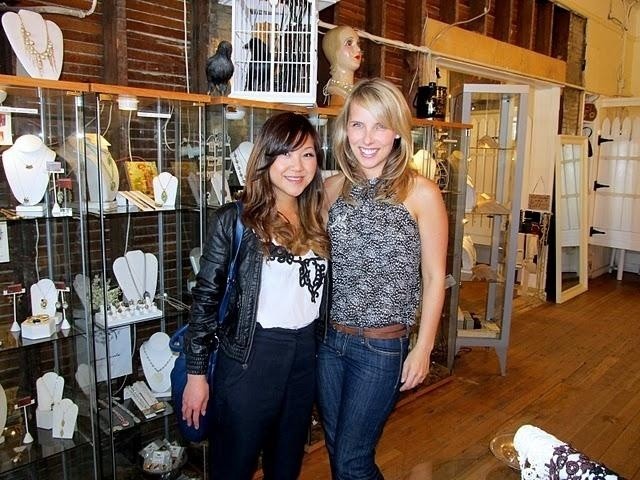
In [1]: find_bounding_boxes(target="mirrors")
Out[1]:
[555,134,590,306]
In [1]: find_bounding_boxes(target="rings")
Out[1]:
[104,299,156,316]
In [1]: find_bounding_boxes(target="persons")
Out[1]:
[312,73,452,480]
[322,24,366,99]
[178,112,334,479]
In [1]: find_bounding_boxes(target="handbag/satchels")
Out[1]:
[169,324,220,441]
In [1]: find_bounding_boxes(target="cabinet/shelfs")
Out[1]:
[206,95,473,479]
[0,71,213,479]
[443,82,530,376]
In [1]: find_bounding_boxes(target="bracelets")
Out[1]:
[26,314,53,326]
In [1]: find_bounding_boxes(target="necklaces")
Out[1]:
[7,143,50,206]
[121,252,149,303]
[55,400,75,440]
[97,377,172,430]
[82,136,119,193]
[41,374,60,411]
[36,283,56,312]
[155,173,175,204]
[15,12,60,77]
[327,75,361,97]
[144,338,177,375]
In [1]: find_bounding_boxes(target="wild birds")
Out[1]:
[242,37,277,91]
[206,40,234,96]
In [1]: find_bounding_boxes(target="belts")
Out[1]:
[329,320,416,339]
